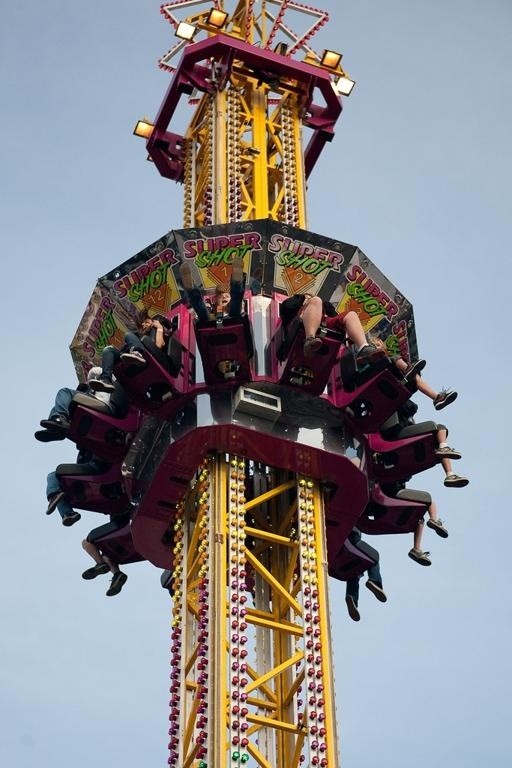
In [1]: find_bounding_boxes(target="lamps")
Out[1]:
[133,119,155,139]
[174,7,356,97]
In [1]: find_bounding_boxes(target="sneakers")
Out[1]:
[35,418,70,442]
[345,595,360,621]
[46,492,81,526]
[232,258,244,283]
[444,475,469,487]
[436,447,461,459]
[180,262,193,290]
[356,346,385,364]
[304,338,322,353]
[434,392,458,410]
[366,580,386,602]
[428,519,449,538]
[404,359,426,379]
[82,563,127,596]
[121,350,146,367]
[408,548,431,565]
[91,377,115,393]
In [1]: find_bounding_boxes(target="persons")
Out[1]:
[34,286,468,622]
